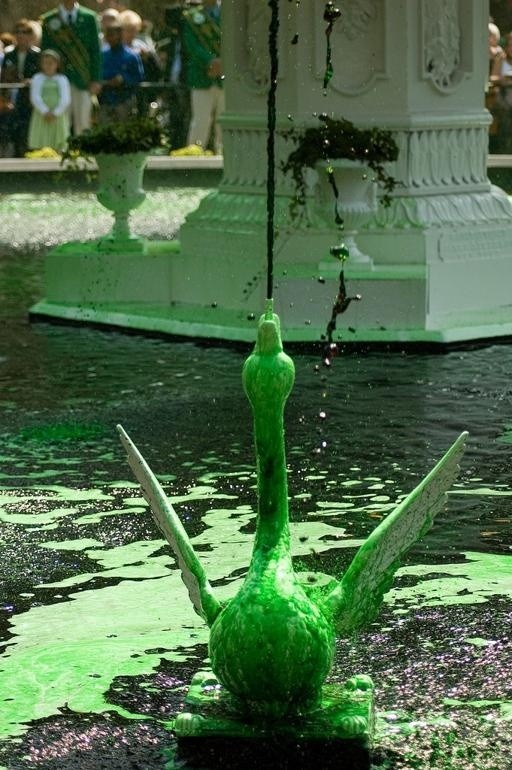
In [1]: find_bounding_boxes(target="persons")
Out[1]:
[487,21,512,155]
[1,0,222,157]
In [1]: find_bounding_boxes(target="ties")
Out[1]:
[68,14,72,27]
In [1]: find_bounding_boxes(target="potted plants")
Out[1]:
[279,112,400,271]
[59,119,168,247]
